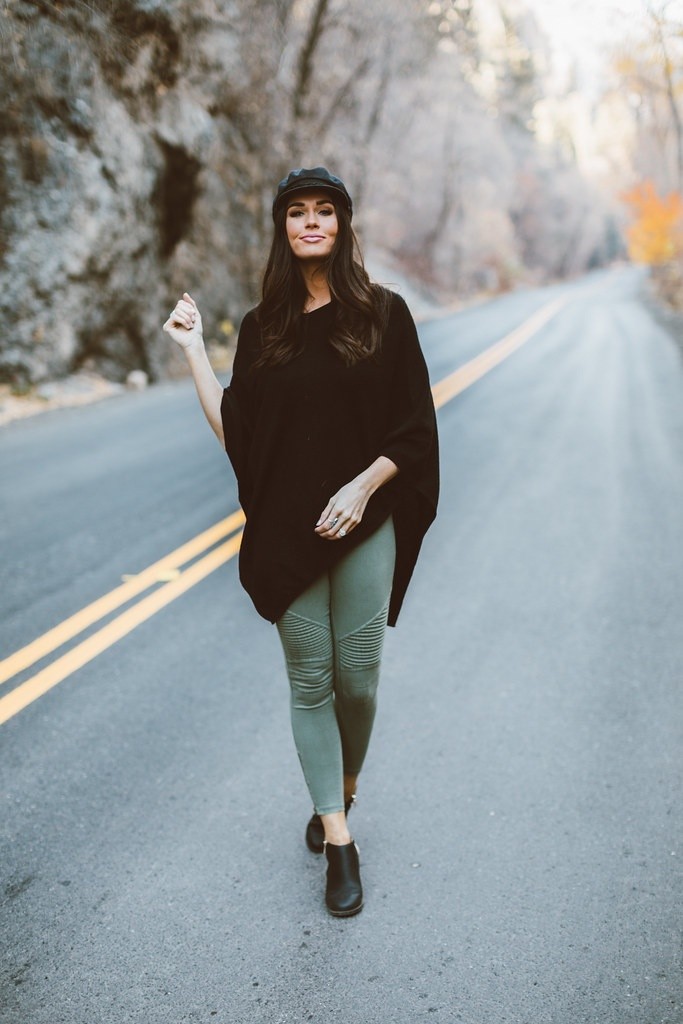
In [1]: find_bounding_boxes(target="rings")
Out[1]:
[338,529,347,537]
[326,517,338,529]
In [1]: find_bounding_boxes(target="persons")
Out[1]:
[160,165,441,917]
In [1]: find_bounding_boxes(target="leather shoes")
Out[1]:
[305,792,365,918]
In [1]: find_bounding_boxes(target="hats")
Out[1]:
[271,167,354,218]
